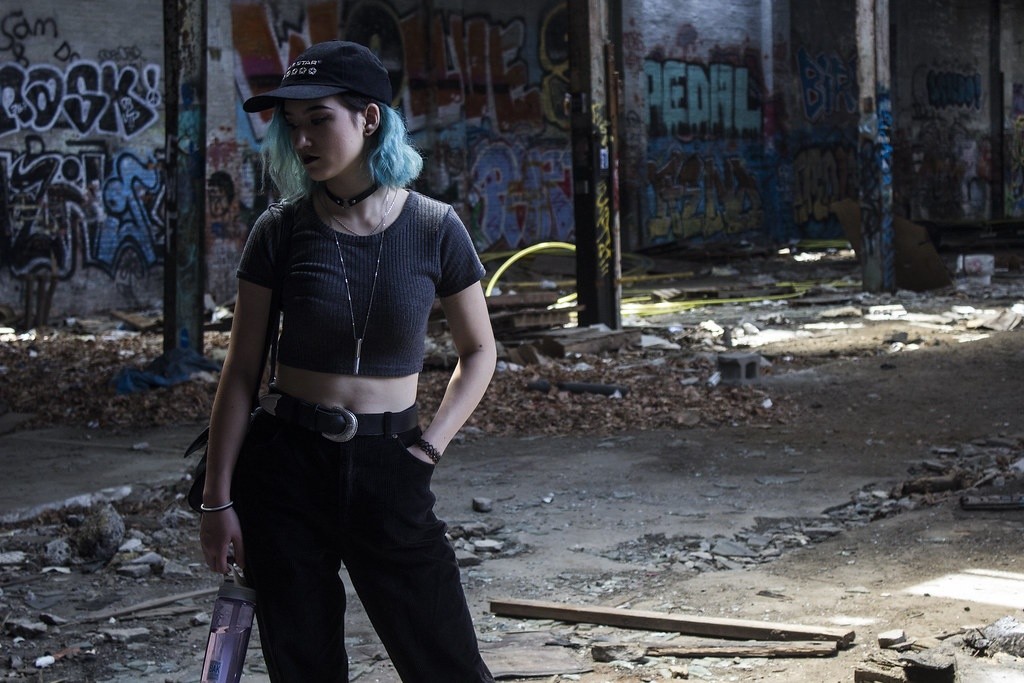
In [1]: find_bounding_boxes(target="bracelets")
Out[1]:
[413,436,441,465]
[199,500,234,513]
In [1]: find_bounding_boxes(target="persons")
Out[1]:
[198,38,502,682]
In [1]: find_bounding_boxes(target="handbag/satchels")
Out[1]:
[183,405,266,514]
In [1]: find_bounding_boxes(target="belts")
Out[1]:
[262,385,418,443]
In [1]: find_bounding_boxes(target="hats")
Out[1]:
[243,38,393,113]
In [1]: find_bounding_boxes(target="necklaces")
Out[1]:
[314,172,401,379]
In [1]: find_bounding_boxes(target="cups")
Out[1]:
[200,563,257,683]
